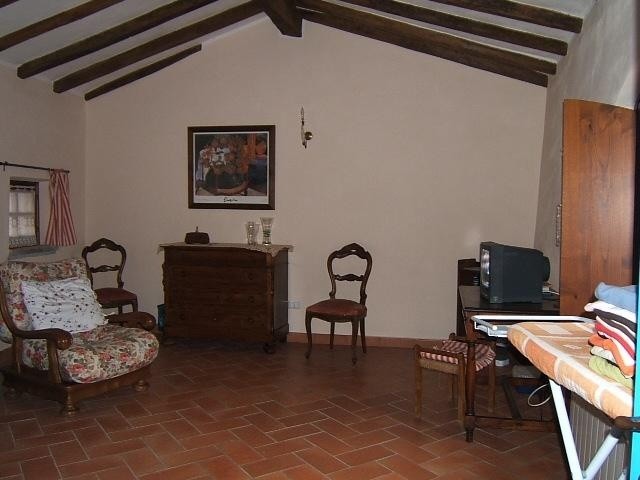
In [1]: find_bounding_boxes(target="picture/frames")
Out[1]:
[188,125,275,210]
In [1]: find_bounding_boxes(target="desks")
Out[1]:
[457,283,561,443]
[104,312,157,332]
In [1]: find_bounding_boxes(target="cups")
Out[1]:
[245,221,259,248]
[259,216,274,248]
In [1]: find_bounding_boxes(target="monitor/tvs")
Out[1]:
[479,242,550,304]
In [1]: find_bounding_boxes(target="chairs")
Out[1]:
[0,255,160,412]
[304,241,372,364]
[81,237,140,314]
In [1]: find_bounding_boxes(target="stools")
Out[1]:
[412,334,496,433]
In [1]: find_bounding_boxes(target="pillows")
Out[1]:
[22,276,108,335]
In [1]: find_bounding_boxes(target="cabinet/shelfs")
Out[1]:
[558,99,640,318]
[507,321,640,479]
[160,242,290,352]
